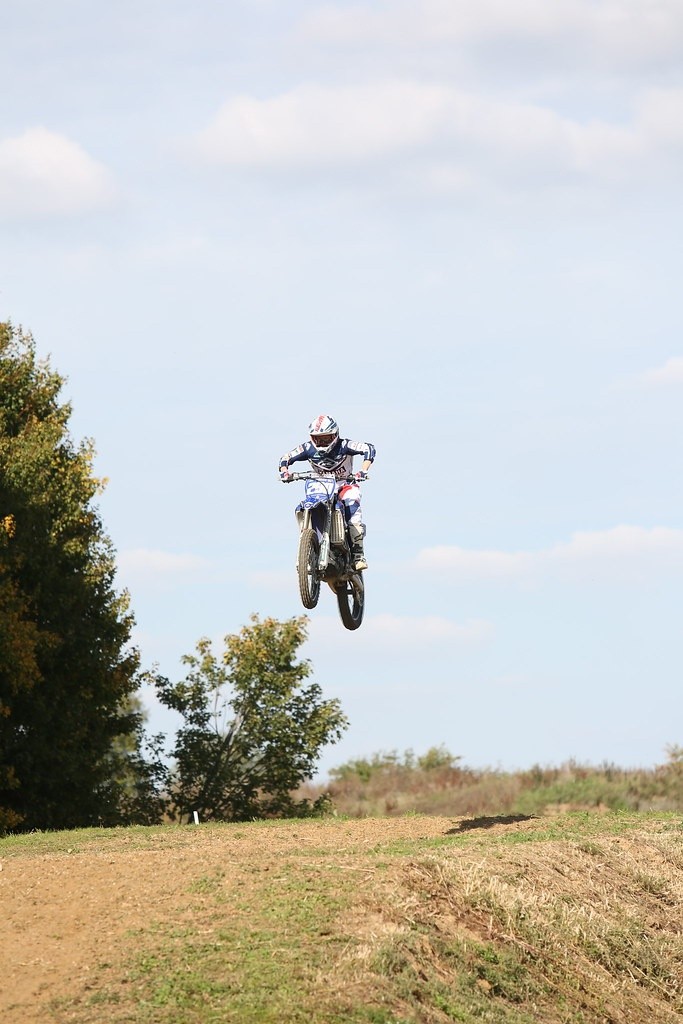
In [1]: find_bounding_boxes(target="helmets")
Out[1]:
[309,415,339,457]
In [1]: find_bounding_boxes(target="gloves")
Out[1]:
[280,470,290,483]
[356,470,368,482]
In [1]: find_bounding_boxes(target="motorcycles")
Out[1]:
[279,471,369,630]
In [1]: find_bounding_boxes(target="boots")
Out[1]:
[349,524,367,571]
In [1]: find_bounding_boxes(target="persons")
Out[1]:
[279,416,375,570]
